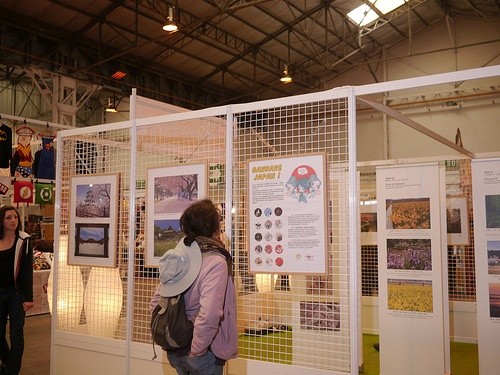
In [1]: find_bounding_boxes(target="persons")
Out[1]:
[150,199,239,375]
[0,205,35,375]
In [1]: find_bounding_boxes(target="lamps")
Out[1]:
[163,8,178,34]
[106,95,116,113]
[280,62,293,85]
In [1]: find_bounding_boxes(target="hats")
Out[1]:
[158,236,203,297]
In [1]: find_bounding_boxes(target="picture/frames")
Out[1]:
[67,152,329,276]
[445,193,472,247]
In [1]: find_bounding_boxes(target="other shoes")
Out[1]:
[0,360,8,375]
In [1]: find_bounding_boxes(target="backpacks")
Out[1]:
[149,295,194,351]
